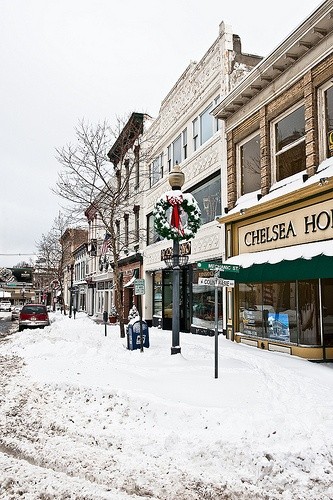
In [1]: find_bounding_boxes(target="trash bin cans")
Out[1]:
[125,319,150,351]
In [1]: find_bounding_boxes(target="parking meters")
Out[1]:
[102,311,108,336]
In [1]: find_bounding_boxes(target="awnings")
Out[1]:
[220,240,333,284]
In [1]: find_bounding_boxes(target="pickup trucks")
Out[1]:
[0,300,12,312]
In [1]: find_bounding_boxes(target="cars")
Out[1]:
[239,304,296,327]
[11,303,53,332]
[154,302,174,319]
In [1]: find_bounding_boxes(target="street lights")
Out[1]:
[167,159,186,354]
[69,260,75,318]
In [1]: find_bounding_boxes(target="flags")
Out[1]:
[102,233,111,253]
